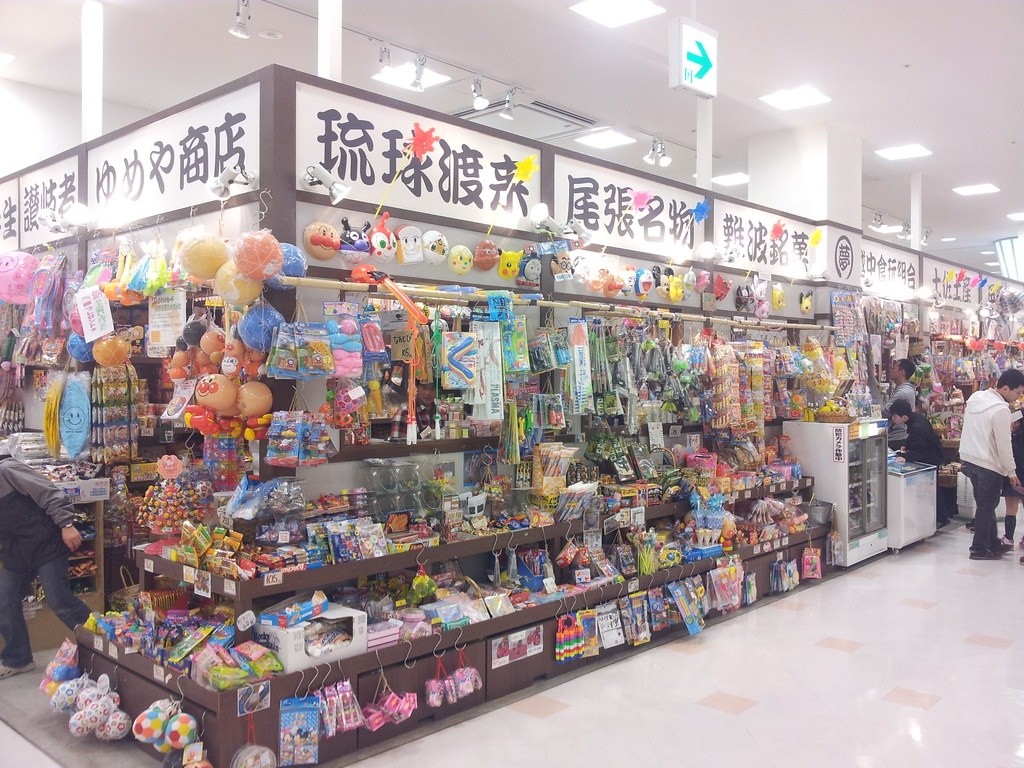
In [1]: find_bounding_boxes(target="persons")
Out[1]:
[882,359,916,459]
[888,399,950,529]
[958,368,1024,566]
[0,454,93,679]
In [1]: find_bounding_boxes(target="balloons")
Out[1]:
[68,687,131,740]
[132,698,198,752]
[50,677,96,715]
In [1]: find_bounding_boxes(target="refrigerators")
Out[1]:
[887,460,937,553]
[783,419,888,567]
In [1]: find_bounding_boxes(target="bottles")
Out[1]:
[851,486,871,509]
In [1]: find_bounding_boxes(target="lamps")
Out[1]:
[530,203,595,247]
[228,0,672,168]
[869,212,930,246]
[862,273,1001,319]
[300,163,351,205]
[207,163,260,197]
[45,217,78,236]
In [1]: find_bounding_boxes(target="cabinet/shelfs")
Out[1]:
[75,476,831,768]
[0,291,223,651]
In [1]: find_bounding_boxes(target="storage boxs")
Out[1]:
[533,443,566,495]
[716,463,802,493]
[656,530,671,541]
[566,441,696,507]
[509,591,529,605]
[251,593,404,675]
[129,463,160,482]
[532,496,559,514]
[419,325,433,385]
[54,479,96,504]
[668,581,706,636]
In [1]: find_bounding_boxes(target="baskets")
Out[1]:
[939,414,964,449]
[927,417,947,438]
[814,396,857,423]
[107,566,191,612]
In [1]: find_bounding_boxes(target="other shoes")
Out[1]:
[1019,536,1024,548]
[0,661,36,680]
[965,523,976,532]
[1000,536,1014,547]
[1019,556,1024,564]
[969,546,1005,560]
[937,516,950,529]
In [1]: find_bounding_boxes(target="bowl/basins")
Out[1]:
[800,501,833,525]
